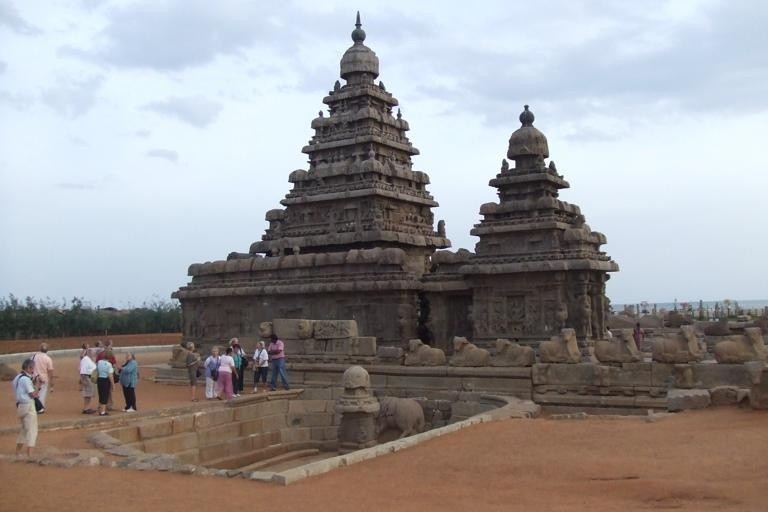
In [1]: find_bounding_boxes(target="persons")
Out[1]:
[605,327,612,338]
[632,322,644,351]
[11,359,42,459]
[29,342,54,408]
[78,334,289,415]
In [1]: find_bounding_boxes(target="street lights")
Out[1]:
[254,362,258,372]
[241,357,249,367]
[91,369,98,384]
[212,369,219,381]
[114,370,120,383]
[196,369,201,377]
[34,398,44,411]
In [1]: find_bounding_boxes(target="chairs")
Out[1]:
[232,389,245,397]
[121,408,137,411]
[208,396,223,399]
[270,385,289,391]
[99,412,108,415]
[82,409,96,414]
